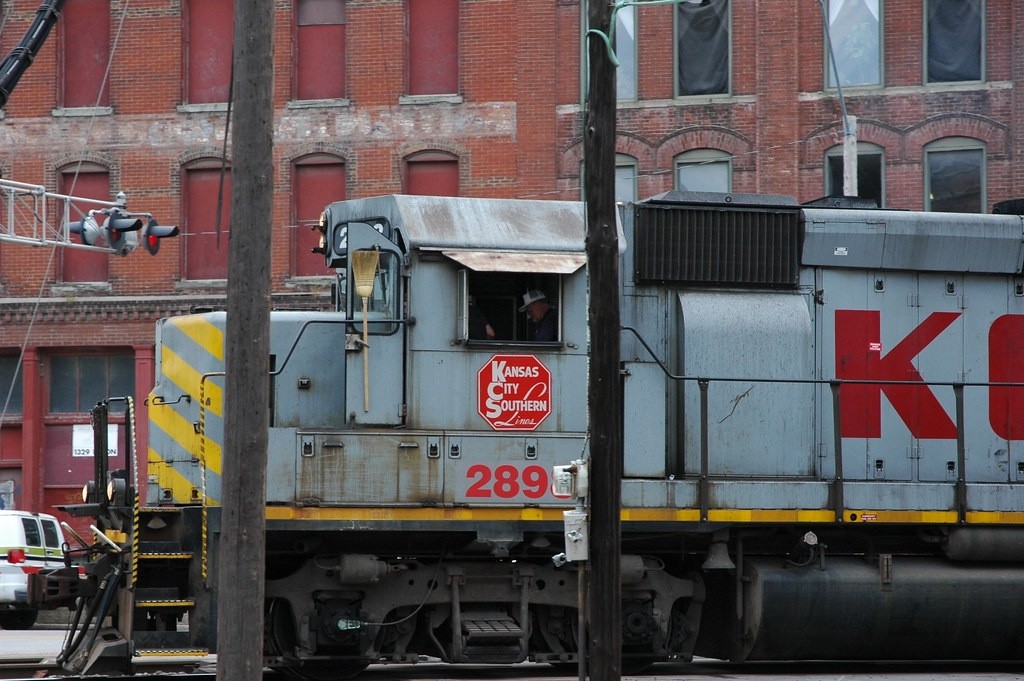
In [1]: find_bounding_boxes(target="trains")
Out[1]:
[35,193,1024,681]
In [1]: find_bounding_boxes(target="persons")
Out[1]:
[519,290,558,341]
[468,306,495,341]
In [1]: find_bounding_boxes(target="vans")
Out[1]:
[0,507,69,631]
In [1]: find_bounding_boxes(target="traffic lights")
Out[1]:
[61,210,101,247]
[102,210,142,252]
[142,218,181,256]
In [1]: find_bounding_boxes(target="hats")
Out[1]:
[519,290,545,312]
